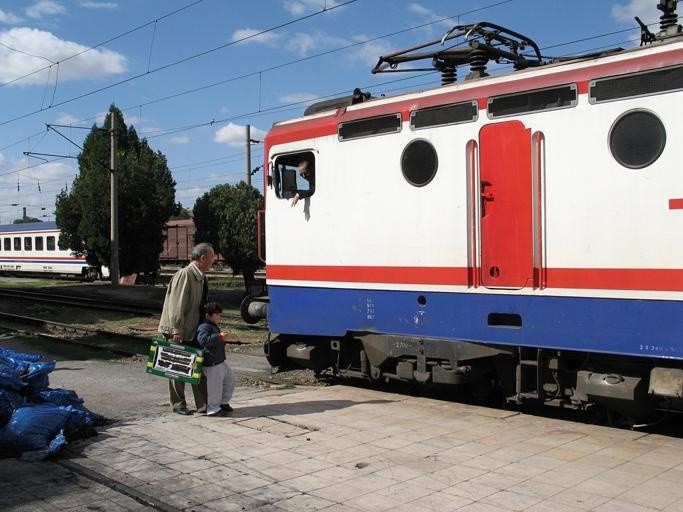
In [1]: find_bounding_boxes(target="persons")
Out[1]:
[156,242,214,415]
[195,301,234,417]
[290,161,313,208]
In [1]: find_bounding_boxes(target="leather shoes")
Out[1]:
[172,407,193,416]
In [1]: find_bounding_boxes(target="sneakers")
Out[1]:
[200,403,235,418]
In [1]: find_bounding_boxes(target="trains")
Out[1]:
[156,219,228,270]
[238,0,682,440]
[0,218,162,281]
